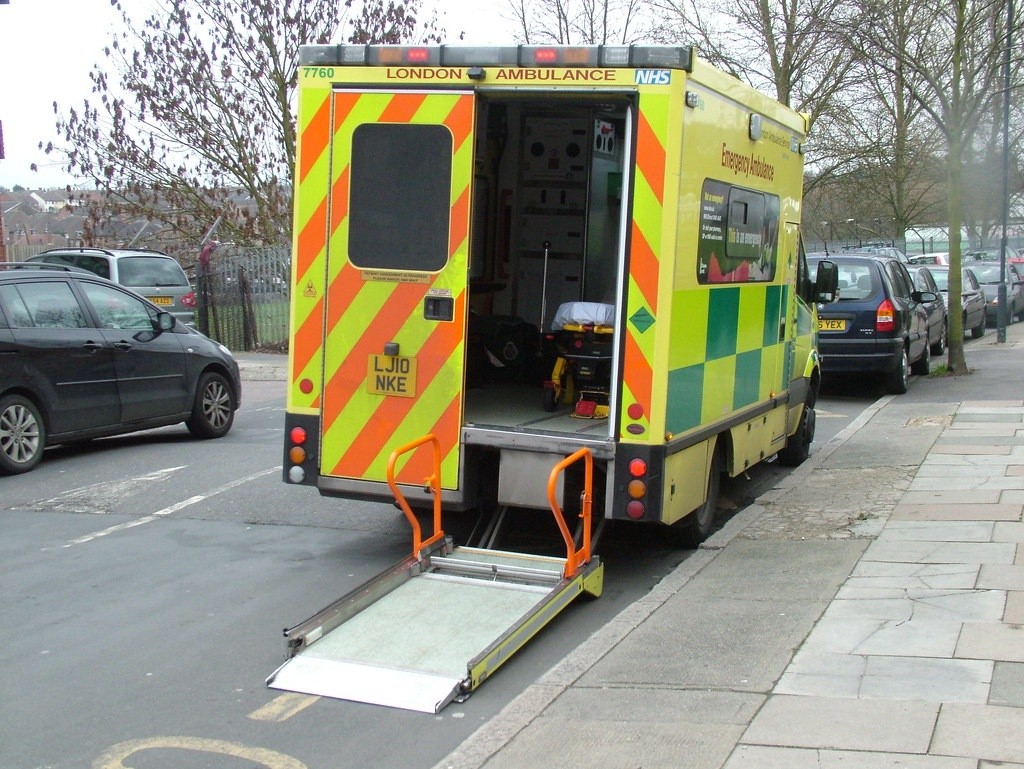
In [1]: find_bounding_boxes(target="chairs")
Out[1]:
[34,297,66,326]
[857,275,873,290]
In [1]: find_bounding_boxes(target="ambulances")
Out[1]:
[277,38,843,553]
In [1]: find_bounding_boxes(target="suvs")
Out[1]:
[0,260,244,477]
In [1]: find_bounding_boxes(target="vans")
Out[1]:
[13,245,199,333]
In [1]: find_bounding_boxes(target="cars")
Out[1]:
[800,251,939,395]
[844,245,1023,356]
